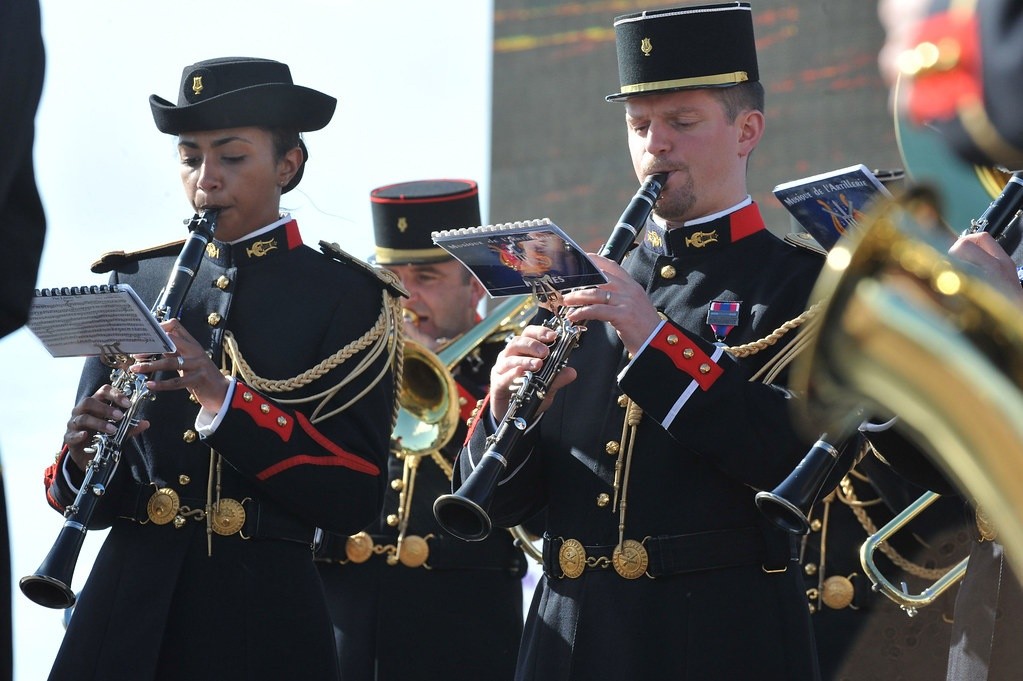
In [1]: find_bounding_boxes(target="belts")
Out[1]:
[333,531,517,575]
[116,483,320,547]
[541,526,783,583]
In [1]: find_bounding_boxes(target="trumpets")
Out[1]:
[381,292,541,459]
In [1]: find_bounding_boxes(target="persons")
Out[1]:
[344,178,543,681]
[794,0,1023,681]
[451,0,823,681]
[43,56,412,681]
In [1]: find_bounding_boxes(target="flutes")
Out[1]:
[753,167,1023,540]
[431,170,669,544]
[17,207,224,610]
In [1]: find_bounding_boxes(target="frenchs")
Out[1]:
[783,184,1023,588]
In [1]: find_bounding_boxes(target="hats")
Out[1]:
[602,1,759,100]
[366,179,480,266]
[148,57,337,136]
